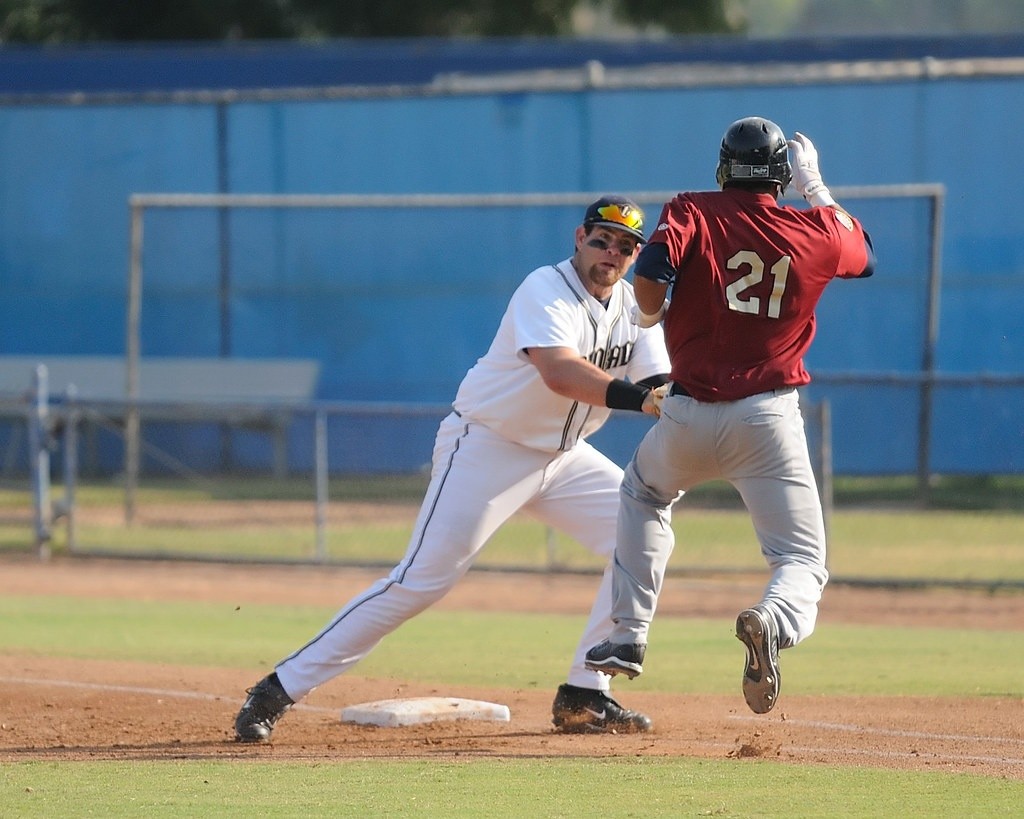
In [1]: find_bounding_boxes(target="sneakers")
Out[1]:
[234,672,297,742]
[550,683,655,734]
[734,603,782,714]
[584,635,647,681]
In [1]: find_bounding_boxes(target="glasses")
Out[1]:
[597,204,644,231]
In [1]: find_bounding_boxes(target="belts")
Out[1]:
[669,381,775,397]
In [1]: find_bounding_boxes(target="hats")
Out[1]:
[583,194,648,244]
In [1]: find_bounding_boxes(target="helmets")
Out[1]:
[715,116,794,197]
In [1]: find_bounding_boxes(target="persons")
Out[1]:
[584,118,875,714]
[234,196,673,734]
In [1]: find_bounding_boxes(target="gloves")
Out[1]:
[787,132,838,207]
[629,297,671,328]
